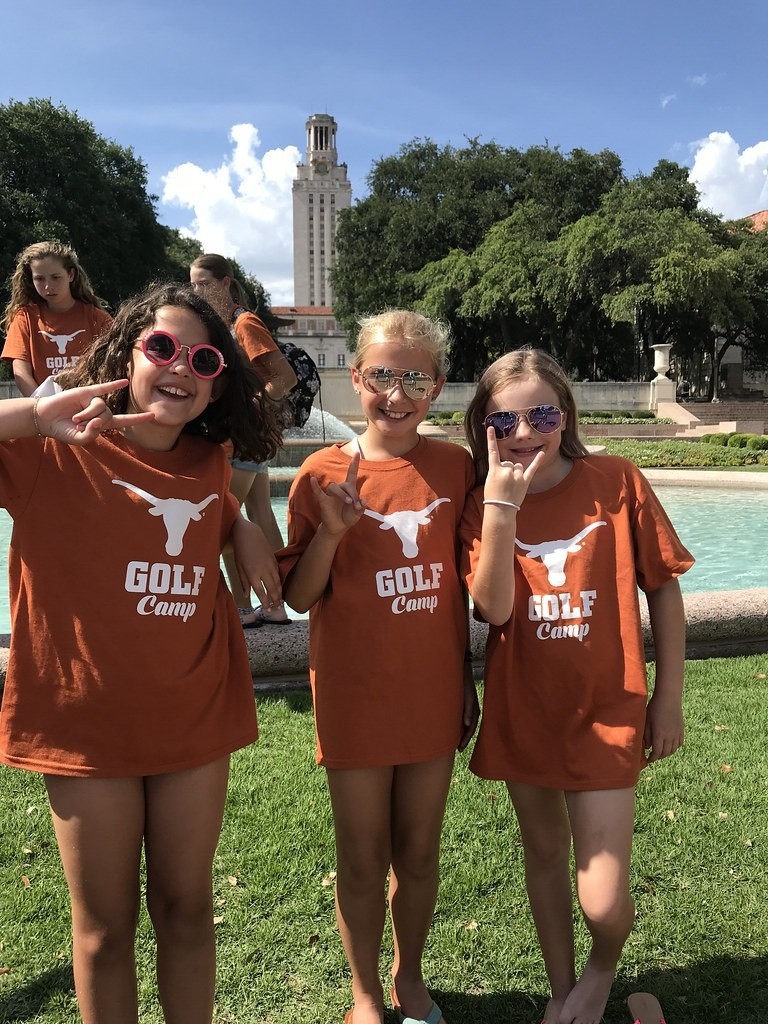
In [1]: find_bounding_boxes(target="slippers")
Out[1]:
[345,1009,354,1024]
[627,993,666,1024]
[391,987,442,1024]
[540,998,552,1024]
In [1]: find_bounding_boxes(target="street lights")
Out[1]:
[592,346,599,382]
[710,321,721,403]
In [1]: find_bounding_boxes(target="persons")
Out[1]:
[273,308,479,1024]
[539,406,548,425]
[455,347,696,1024]
[1,241,299,628]
[487,412,516,437]
[0,285,280,1024]
[409,373,416,391]
[383,369,391,389]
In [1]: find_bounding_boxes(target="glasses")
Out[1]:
[129,331,228,379]
[357,365,438,402]
[481,404,564,441]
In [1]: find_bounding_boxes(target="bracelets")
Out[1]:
[32,396,42,437]
[482,499,520,512]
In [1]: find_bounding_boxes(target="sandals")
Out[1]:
[255,601,292,626]
[237,607,263,628]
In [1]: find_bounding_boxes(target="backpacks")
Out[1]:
[231,307,322,428]
